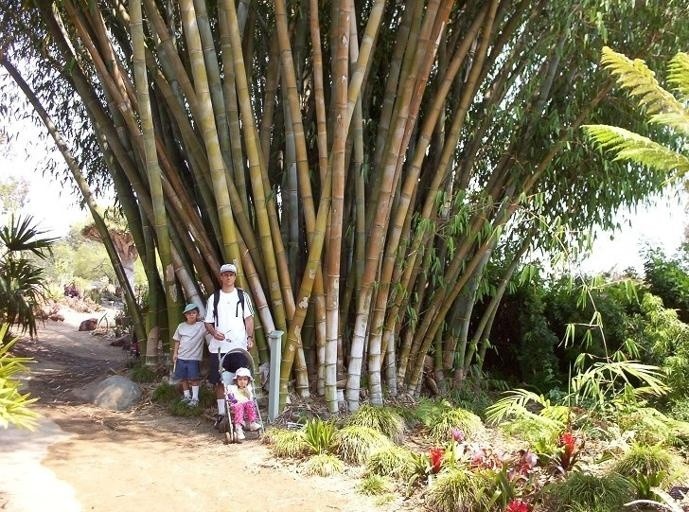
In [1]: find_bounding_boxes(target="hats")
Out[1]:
[183,304,198,314]
[219,263,238,275]
[232,367,253,381]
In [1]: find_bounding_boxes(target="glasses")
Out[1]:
[221,328,234,343]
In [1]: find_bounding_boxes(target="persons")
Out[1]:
[226,367,262,439]
[172,264,255,425]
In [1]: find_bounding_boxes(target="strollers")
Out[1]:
[218,347,265,444]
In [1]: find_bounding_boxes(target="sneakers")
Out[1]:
[235,428,245,440]
[249,421,261,432]
[188,398,200,407]
[214,413,225,427]
[181,395,192,401]
